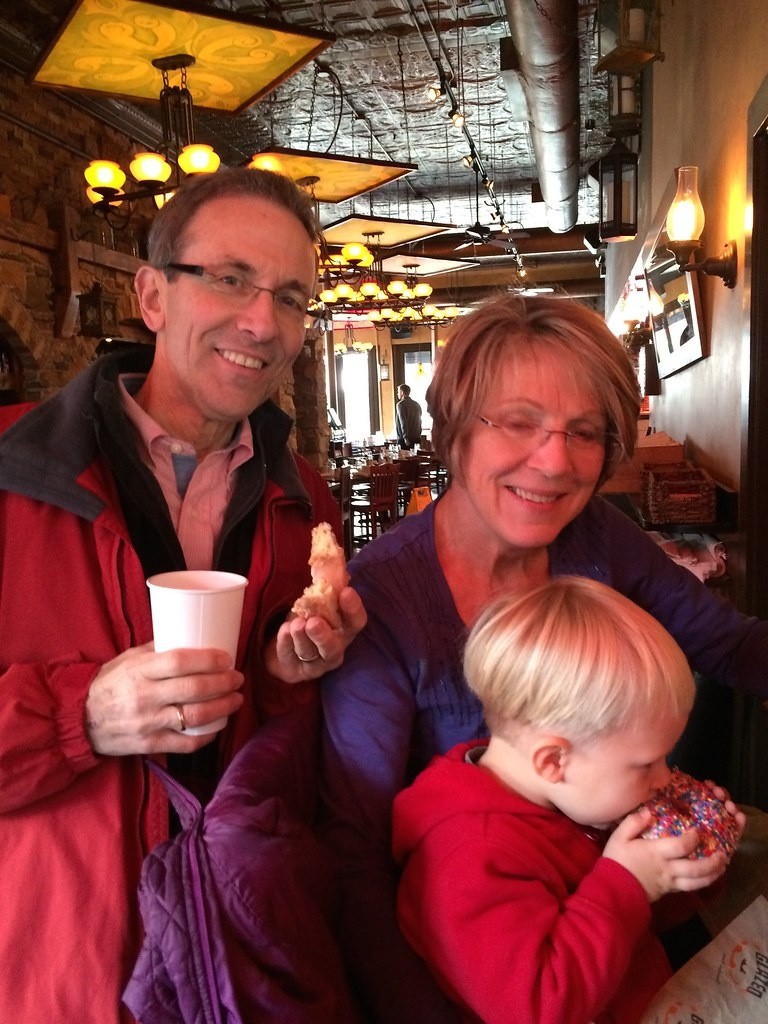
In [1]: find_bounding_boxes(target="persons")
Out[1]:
[395,384,422,451]
[321,296,768,1024]
[0,164,368,1024]
[392,574,747,1024]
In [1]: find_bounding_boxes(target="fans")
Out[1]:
[453,161,530,251]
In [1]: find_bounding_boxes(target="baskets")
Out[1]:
[639,458,718,526]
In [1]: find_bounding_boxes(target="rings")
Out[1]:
[178,705,187,731]
[296,652,319,665]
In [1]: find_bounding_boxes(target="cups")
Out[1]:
[146,569,249,738]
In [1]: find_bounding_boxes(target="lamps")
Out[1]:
[448,105,465,128]
[84,54,220,230]
[482,175,494,189]
[462,144,477,166]
[428,82,446,102]
[295,176,459,332]
[666,165,738,289]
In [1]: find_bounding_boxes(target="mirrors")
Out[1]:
[643,217,710,380]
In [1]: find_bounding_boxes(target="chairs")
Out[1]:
[334,450,447,563]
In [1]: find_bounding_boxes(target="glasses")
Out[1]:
[165,261,315,327]
[465,406,626,469]
